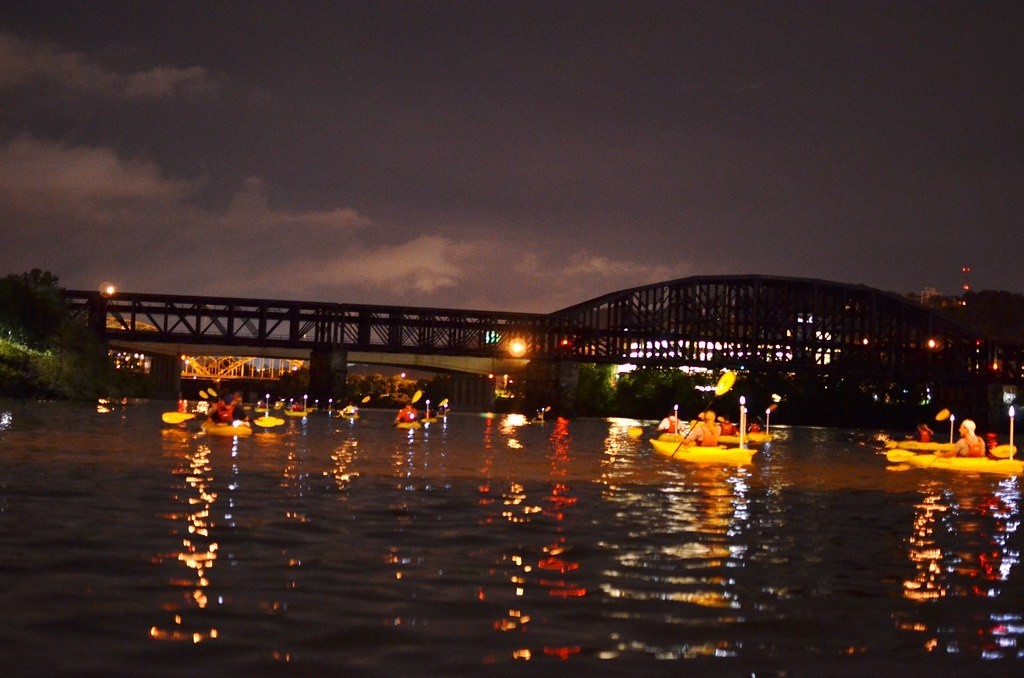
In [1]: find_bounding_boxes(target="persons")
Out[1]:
[906,423,932,445]
[207,390,448,425]
[937,420,988,459]
[658,408,764,449]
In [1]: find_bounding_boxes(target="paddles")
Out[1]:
[361,396,371,404]
[886,444,1018,463]
[717,416,724,423]
[199,390,209,399]
[671,371,739,457]
[545,406,551,413]
[439,398,449,408]
[886,408,950,450]
[207,387,218,398]
[626,426,652,439]
[411,390,422,404]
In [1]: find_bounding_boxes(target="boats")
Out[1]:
[392,399,422,430]
[200,391,253,438]
[338,401,360,420]
[649,395,757,467]
[884,414,960,456]
[435,401,448,422]
[714,407,773,446]
[886,405,1024,479]
[165,392,208,427]
[420,399,436,427]
[626,403,693,449]
[252,392,333,431]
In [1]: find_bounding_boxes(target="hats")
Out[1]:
[963,420,976,436]
[699,411,715,421]
[225,394,233,403]
